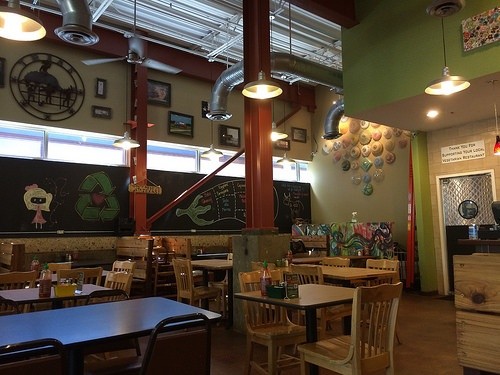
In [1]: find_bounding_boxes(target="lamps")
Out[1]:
[493,103,500,156]
[0,0,46,42]
[425,16,470,96]
[243,0,283,100]
[271,14,288,142]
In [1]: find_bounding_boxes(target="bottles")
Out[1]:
[38,262,52,298]
[31,255,39,276]
[282,250,293,268]
[261,261,272,296]
[357,242,370,256]
[468,217,479,240]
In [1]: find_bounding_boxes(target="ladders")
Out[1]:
[406,143,420,293]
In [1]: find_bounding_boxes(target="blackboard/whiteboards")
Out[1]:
[0,156,311,239]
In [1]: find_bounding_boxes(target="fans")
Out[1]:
[83,0,182,75]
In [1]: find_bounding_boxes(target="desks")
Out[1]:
[308,264,397,335]
[0,296,222,375]
[292,256,375,265]
[456,239,500,254]
[234,284,354,375]
[0,283,113,308]
[46,257,119,269]
[192,259,234,327]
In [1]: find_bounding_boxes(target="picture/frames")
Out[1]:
[291,127,306,142]
[146,78,172,107]
[167,111,194,138]
[219,124,240,146]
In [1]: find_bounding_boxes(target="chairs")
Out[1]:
[0,256,401,375]
